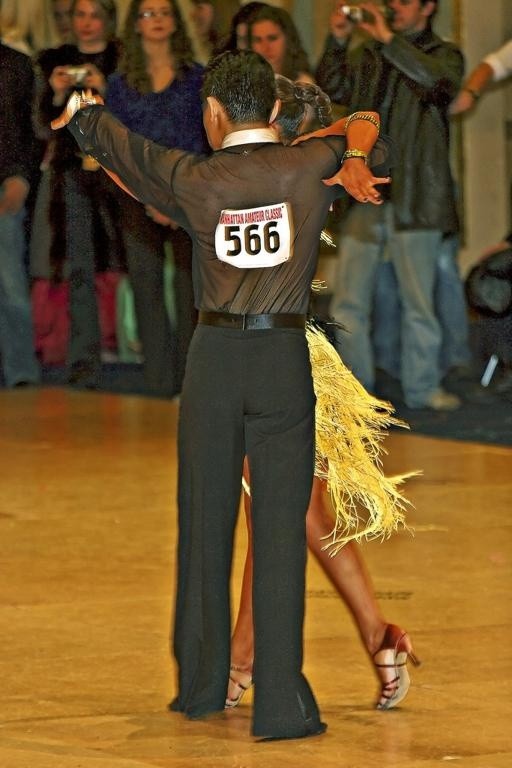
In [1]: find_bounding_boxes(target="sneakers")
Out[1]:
[404,386,463,413]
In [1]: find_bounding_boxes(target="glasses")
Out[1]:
[136,10,174,18]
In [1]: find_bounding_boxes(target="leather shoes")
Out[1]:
[168,692,216,720]
[257,720,329,739]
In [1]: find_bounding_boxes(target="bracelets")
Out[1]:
[345,114,381,138]
[340,148,368,165]
[463,87,480,100]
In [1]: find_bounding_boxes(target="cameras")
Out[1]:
[62,66,88,89]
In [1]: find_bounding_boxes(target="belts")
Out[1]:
[196,311,308,332]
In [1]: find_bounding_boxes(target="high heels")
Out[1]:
[218,659,257,709]
[370,623,421,713]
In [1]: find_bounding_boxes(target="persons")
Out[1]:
[50,91,403,744]
[1,1,511,414]
[78,72,422,710]
